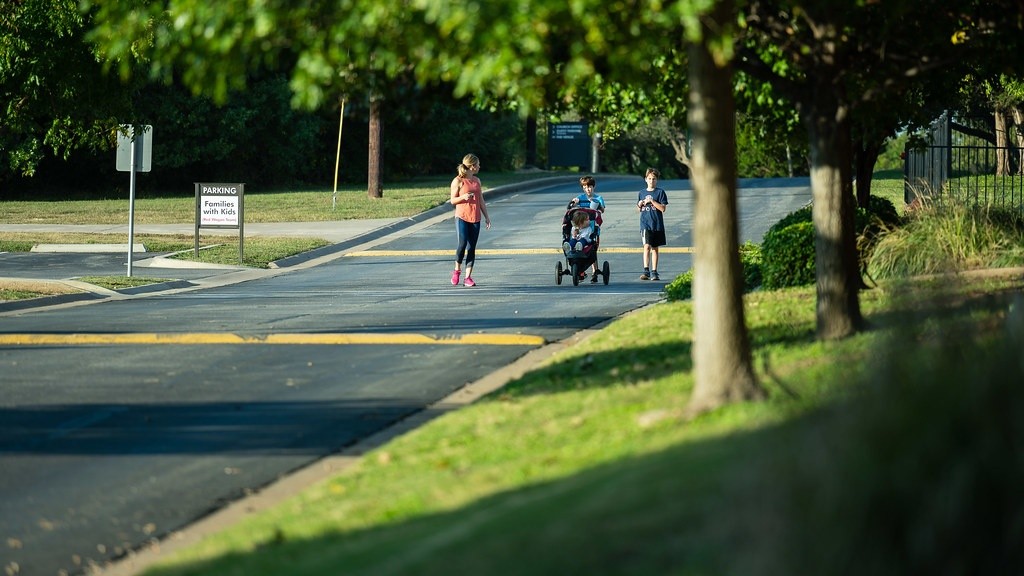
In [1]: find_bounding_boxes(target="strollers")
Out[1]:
[555,199,611,287]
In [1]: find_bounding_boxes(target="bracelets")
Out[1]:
[637,205,641,208]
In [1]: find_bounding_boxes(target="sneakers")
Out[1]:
[640,271,650,280]
[650,272,660,281]
[462,276,476,287]
[451,269,462,285]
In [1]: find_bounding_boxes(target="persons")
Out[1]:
[563,210,600,253]
[637,167,668,280]
[450,153,491,287]
[572,176,606,283]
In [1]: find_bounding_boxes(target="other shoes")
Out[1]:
[575,242,583,251]
[563,242,571,255]
[579,272,588,281]
[590,275,598,284]
